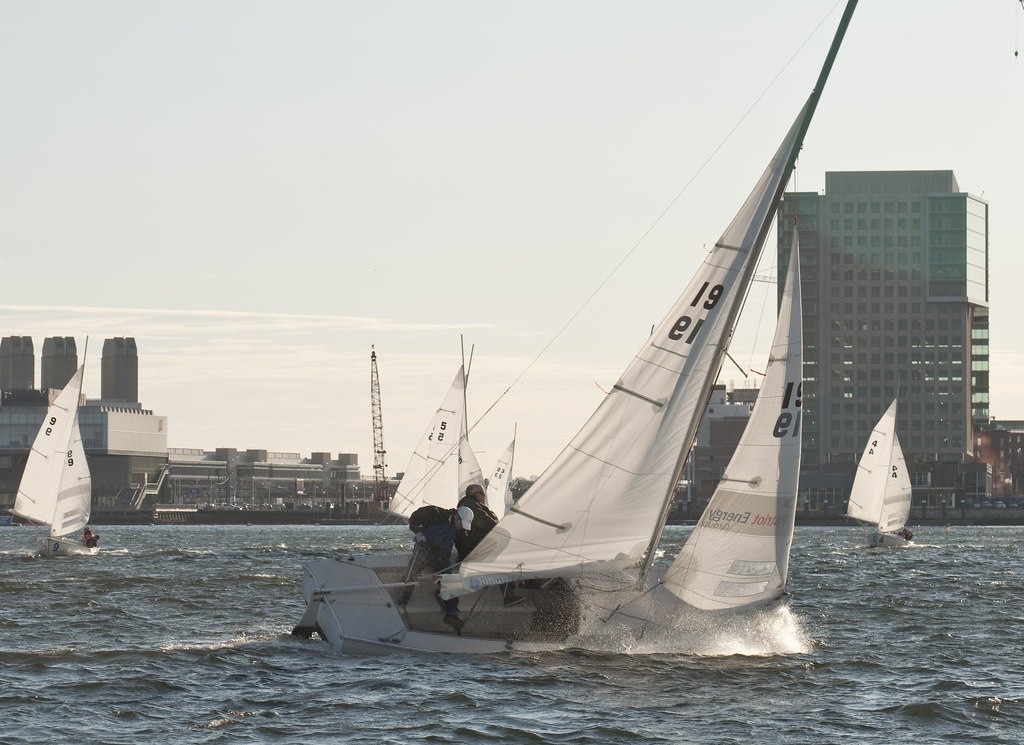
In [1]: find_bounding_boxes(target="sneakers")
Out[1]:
[503,595,526,607]
[443,615,465,629]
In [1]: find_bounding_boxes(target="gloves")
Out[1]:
[415,532,427,543]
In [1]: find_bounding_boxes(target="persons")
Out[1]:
[902,528,912,541]
[83,526,94,542]
[398,505,474,624]
[455,483,512,563]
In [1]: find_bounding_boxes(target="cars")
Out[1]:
[982,501,1018,509]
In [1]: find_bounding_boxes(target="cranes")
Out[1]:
[370,343,386,483]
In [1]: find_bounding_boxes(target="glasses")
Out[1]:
[474,491,485,495]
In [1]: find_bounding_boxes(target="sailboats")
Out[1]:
[8,335,101,558]
[290,0,859,672]
[847,373,914,549]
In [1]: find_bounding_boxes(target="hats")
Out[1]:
[457,506,475,531]
